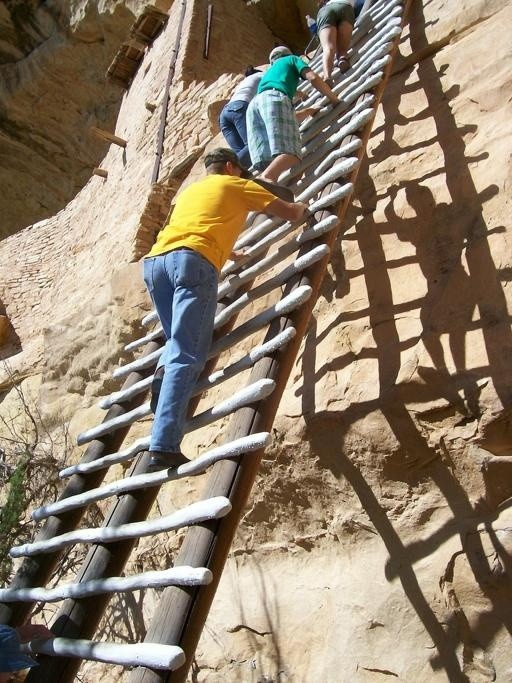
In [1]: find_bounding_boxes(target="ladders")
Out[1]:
[0,0,412,683]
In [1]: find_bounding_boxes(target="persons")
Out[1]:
[317,0,359,89]
[0,619,53,683]
[220,64,266,169]
[143,147,308,474]
[245,45,342,204]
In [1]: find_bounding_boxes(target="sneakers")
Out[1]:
[151,367,164,416]
[321,77,334,90]
[147,448,206,477]
[337,57,352,75]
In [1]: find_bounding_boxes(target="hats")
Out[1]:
[203,147,250,179]
[268,46,293,65]
[0,624,41,674]
